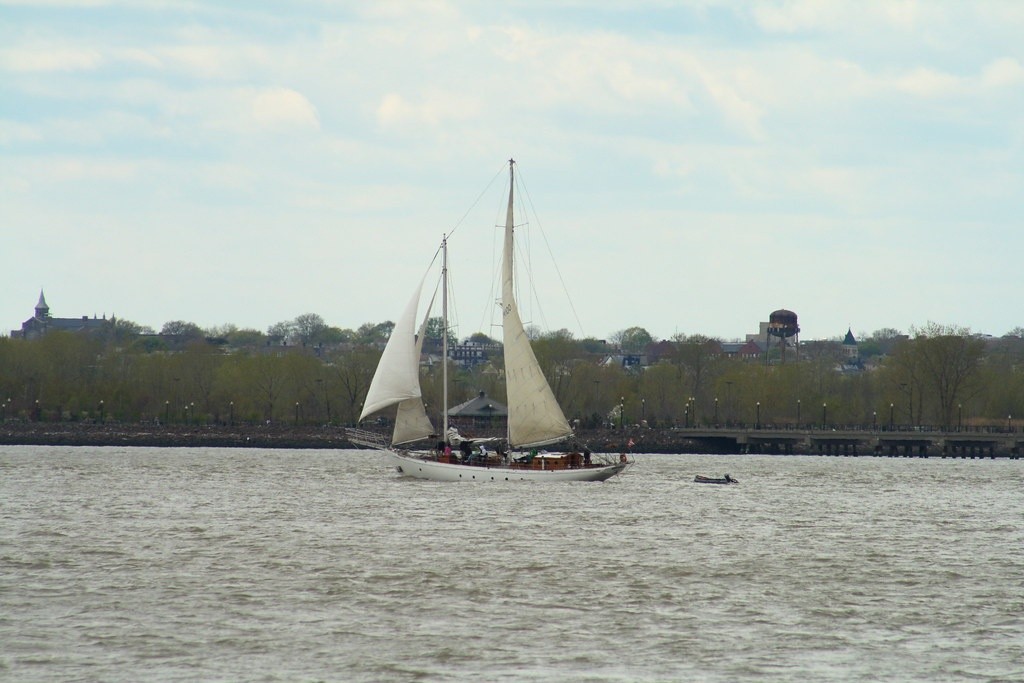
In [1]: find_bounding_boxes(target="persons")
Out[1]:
[259,418,271,428]
[154,417,159,426]
[464,445,548,466]
[445,444,451,455]
[657,421,1019,432]
[584,444,590,464]
[322,417,391,431]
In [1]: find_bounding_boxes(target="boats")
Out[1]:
[694,472,739,485]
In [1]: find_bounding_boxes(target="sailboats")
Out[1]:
[342,157,637,483]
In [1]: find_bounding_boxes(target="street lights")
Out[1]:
[35,400,39,418]
[756,401,760,423]
[165,400,169,424]
[686,403,690,428]
[2,404,6,419]
[185,405,188,426]
[1007,415,1012,433]
[873,412,876,432]
[714,397,718,422]
[190,402,195,425]
[641,398,646,420]
[621,396,624,405]
[100,400,104,422]
[359,403,363,423]
[958,404,962,432]
[822,403,827,431]
[229,401,234,422]
[688,397,692,413]
[797,399,801,430]
[692,397,695,419]
[295,402,299,422]
[620,403,624,427]
[890,403,894,431]
